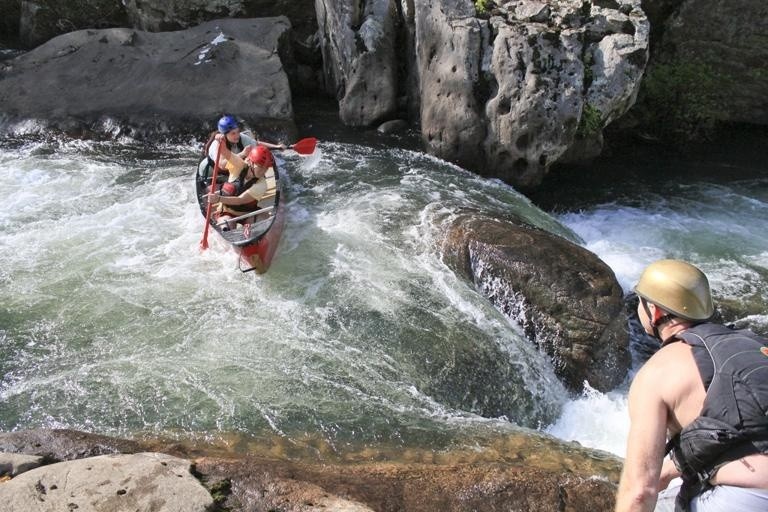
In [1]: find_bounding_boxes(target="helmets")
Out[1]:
[633,260,716,323]
[217,116,239,133]
[249,145,273,167]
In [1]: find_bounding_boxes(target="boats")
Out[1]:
[193,114,282,277]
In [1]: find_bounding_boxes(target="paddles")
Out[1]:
[200,140,223,251]
[269,137,316,154]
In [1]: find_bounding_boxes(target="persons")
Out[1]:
[198,115,286,231]
[615,259,768,512]
